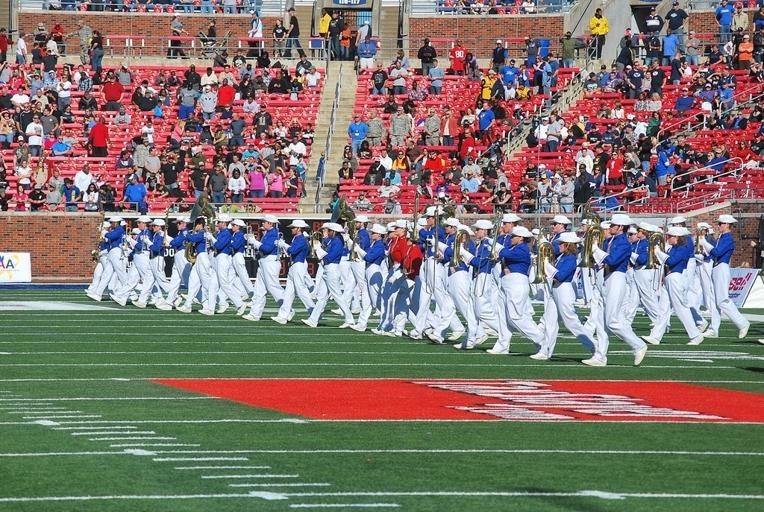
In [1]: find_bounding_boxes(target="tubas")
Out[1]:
[531,222,664,299]
[447,230,471,269]
[183,192,216,263]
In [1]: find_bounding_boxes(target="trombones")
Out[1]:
[424,193,438,295]
[473,207,504,297]
[402,190,421,276]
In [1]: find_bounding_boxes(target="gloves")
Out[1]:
[484,235,505,258]
[427,234,448,253]
[450,242,474,264]
[580,236,608,266]
[123,235,137,249]
[243,233,262,250]
[122,242,133,257]
[273,238,291,251]
[101,229,108,241]
[309,239,327,260]
[698,237,714,254]
[341,226,352,251]
[354,243,366,258]
[91,248,108,257]
[693,251,704,265]
[651,242,674,266]
[206,232,217,244]
[528,251,559,281]
[630,251,640,264]
[159,231,173,245]
[140,235,153,250]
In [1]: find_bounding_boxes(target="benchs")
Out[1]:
[433,1,576,17]
[47,0,261,16]
[0,64,324,213]
[338,64,760,213]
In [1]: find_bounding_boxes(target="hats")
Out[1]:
[260,214,281,223]
[369,223,387,235]
[387,219,474,241]
[285,219,309,229]
[549,216,571,225]
[107,215,248,227]
[554,232,582,244]
[500,213,522,222]
[669,216,688,224]
[715,214,739,224]
[318,222,343,233]
[512,225,532,239]
[471,219,493,230]
[531,228,544,235]
[422,206,444,217]
[353,215,371,223]
[610,214,633,225]
[581,218,693,236]
[130,228,142,235]
[697,221,716,236]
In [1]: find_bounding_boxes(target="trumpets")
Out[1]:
[696,224,709,254]
[123,206,175,254]
[242,219,362,264]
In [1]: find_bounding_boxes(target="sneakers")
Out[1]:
[581,358,608,368]
[242,314,260,322]
[448,329,463,341]
[703,330,719,339]
[641,335,661,346]
[486,348,509,354]
[529,352,549,361]
[371,327,445,344]
[452,334,489,350]
[686,334,704,346]
[739,323,750,338]
[339,321,354,329]
[530,297,680,318]
[83,288,231,316]
[236,300,252,316]
[697,317,708,333]
[633,345,646,366]
[309,291,381,317]
[350,325,367,332]
[270,308,296,326]
[301,318,317,327]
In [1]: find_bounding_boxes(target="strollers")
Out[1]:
[175,28,231,59]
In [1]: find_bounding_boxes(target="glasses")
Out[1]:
[372,232,378,234]
[476,228,480,231]
